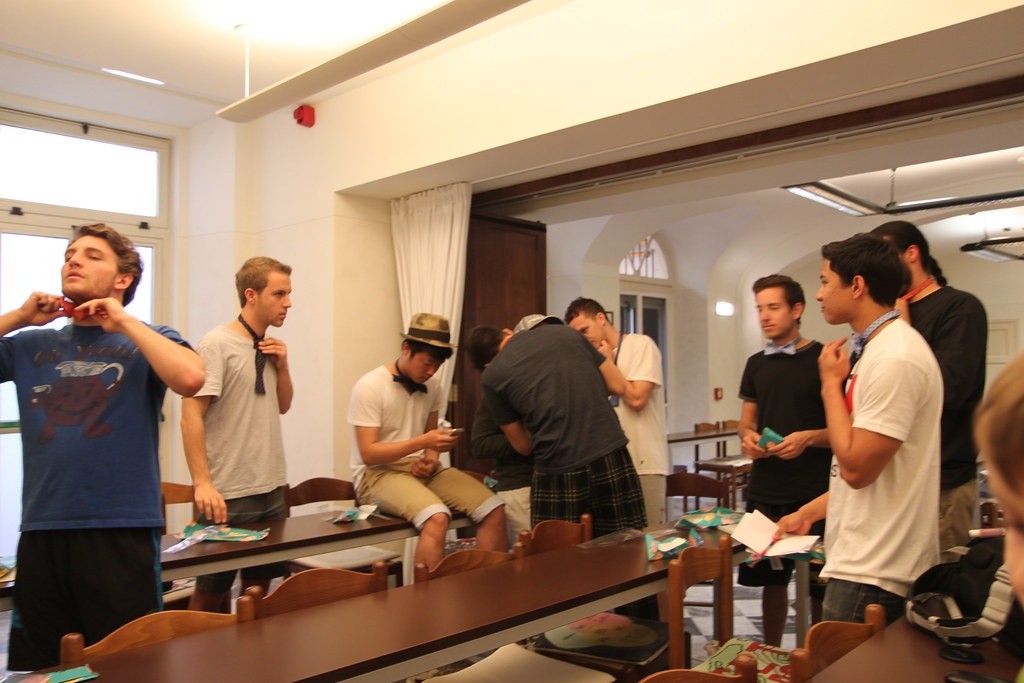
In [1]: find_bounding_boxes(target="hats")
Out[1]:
[513,314,564,330]
[400,312,458,349]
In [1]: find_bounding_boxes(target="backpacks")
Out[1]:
[907,534,1024,662]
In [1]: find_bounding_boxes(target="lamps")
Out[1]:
[784,167,1024,261]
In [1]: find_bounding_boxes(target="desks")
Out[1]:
[665,429,741,512]
[0,507,471,614]
[698,455,753,512]
[0,517,812,683]
[815,617,1024,683]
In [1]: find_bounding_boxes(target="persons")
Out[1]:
[816,221,1024,624]
[738,274,833,647]
[0,223,205,672]
[469,298,674,622]
[348,313,509,571]
[180,256,293,612]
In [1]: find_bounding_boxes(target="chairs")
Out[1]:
[0,419,1001,683]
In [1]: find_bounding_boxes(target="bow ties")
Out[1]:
[391,358,429,394]
[238,314,265,395]
[763,335,802,356]
[851,310,903,355]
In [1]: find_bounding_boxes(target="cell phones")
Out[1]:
[450,428,464,436]
